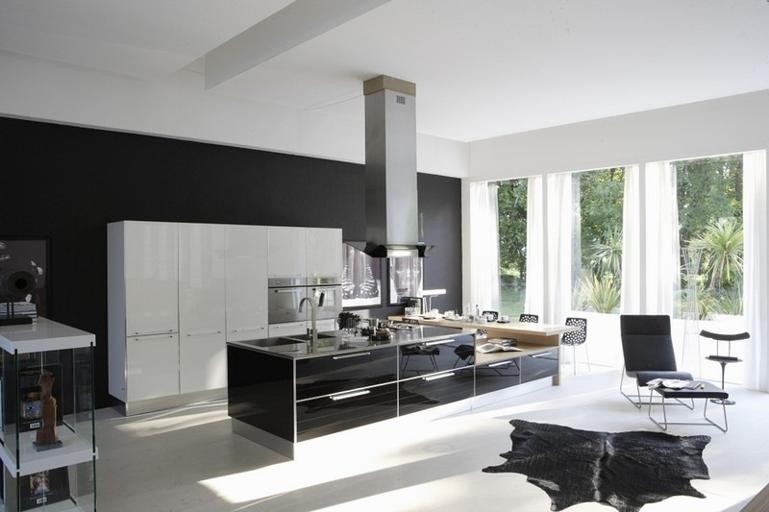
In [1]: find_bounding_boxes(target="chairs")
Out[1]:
[560,317,587,375]
[699,329,750,405]
[519,314,538,323]
[619,314,695,410]
[482,311,498,320]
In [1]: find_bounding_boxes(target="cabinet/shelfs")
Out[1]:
[0,315,97,512]
[106,220,343,417]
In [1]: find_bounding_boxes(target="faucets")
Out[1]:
[299,297,318,353]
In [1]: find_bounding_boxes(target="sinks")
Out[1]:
[286,332,342,347]
[234,336,308,353]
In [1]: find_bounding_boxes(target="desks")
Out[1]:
[648,381,729,433]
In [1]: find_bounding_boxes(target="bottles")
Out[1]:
[20,391,41,421]
[475,304,479,318]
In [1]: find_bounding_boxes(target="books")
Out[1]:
[0,301,37,323]
[476,343,522,354]
[488,336,519,346]
[646,378,689,390]
[685,381,701,390]
